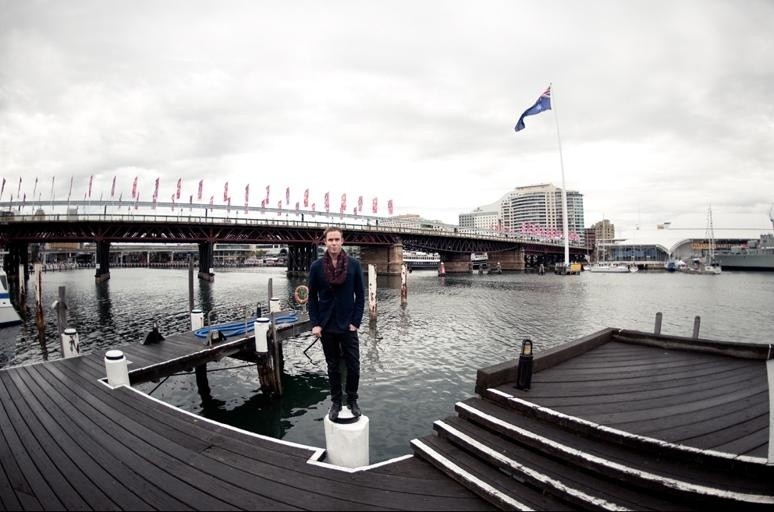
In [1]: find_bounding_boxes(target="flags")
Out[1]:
[2,176,395,227]
[513,86,552,133]
[520,220,581,246]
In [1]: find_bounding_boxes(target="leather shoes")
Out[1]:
[329,400,342,421]
[347,397,361,418]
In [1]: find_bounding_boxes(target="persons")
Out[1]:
[305,226,366,421]
[527,252,536,267]
[523,254,529,268]
[536,256,545,275]
[495,261,501,271]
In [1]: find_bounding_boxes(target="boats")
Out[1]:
[590,260,639,273]
[663,256,690,272]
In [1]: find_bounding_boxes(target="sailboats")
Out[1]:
[691,197,721,275]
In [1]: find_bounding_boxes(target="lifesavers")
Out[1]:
[295,286,309,305]
